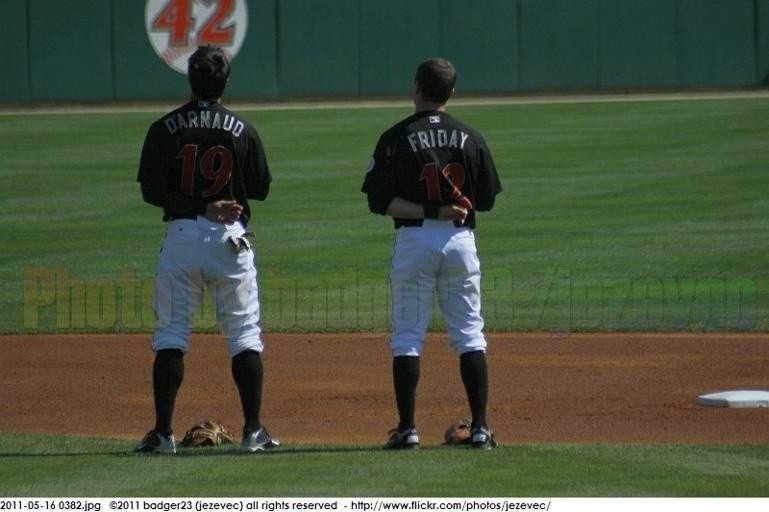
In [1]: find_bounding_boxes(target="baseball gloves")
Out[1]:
[183,421,233,444]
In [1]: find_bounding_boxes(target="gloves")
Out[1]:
[229,231,256,252]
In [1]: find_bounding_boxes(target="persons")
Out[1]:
[134,45,283,453]
[361,59,502,450]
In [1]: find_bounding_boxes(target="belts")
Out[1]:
[395,218,469,227]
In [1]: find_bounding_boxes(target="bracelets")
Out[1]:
[422,201,440,220]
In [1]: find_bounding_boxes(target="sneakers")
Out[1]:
[134,430,177,454]
[383,427,420,450]
[241,425,281,451]
[471,424,499,449]
